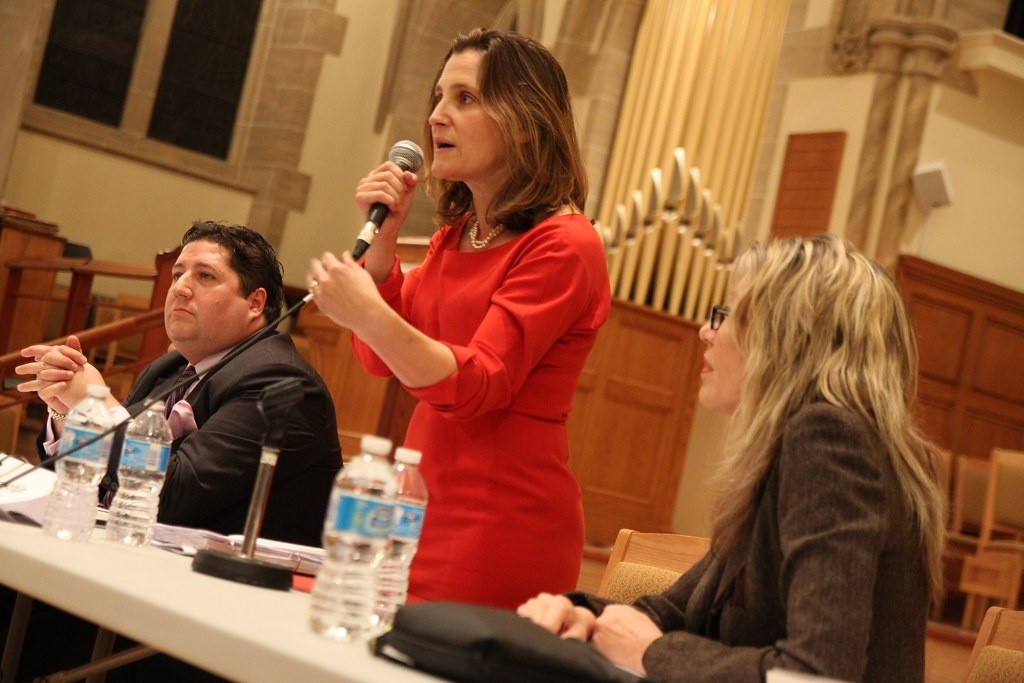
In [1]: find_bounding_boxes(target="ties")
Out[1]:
[163,366,199,420]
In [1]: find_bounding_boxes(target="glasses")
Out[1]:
[710,304,730,329]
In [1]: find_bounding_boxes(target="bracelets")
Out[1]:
[46,406,66,422]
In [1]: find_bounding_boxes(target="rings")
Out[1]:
[308,279,318,292]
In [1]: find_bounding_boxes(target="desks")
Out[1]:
[0,508,446,683]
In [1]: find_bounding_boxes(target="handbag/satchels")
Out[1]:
[368,602,644,683]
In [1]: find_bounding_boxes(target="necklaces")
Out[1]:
[468,219,505,249]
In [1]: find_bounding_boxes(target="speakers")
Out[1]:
[912,161,952,213]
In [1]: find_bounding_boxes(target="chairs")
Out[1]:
[936,447,1024,683]
[599,527,713,603]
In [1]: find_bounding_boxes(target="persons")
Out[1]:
[16,220,344,547]
[518,233,945,683]
[307,26,612,611]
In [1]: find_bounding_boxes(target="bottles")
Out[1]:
[310,435,398,639]
[105,400,173,546]
[43,384,115,542]
[376,448,428,628]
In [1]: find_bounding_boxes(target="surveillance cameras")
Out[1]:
[353,140,424,259]
